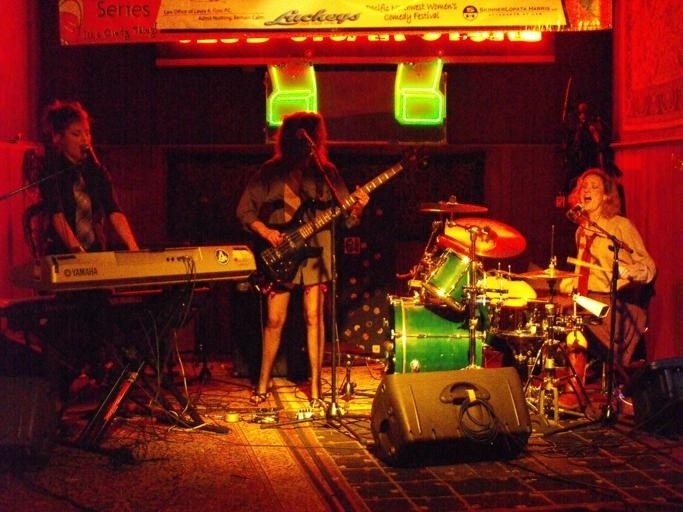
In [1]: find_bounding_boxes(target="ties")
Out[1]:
[577,233,597,309]
[74,169,95,251]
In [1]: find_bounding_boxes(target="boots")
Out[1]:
[558,346,585,409]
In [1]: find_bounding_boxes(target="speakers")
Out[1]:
[623,356,683,430]
[371,366,532,468]
[0,375,58,472]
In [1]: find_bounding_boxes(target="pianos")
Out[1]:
[32,244,256,295]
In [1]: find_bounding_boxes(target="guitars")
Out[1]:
[253,146,424,294]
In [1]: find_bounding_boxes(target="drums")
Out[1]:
[422,248,487,312]
[472,274,538,331]
[384,296,487,374]
[495,302,554,337]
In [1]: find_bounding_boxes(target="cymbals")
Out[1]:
[519,270,583,277]
[415,202,488,216]
[444,216,527,259]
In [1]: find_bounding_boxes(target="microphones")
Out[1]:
[568,202,585,217]
[296,128,314,147]
[82,144,101,170]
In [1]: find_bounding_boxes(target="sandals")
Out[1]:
[311,396,323,410]
[252,391,269,404]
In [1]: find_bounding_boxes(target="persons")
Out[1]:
[574,168,658,399]
[233,113,370,409]
[38,101,171,396]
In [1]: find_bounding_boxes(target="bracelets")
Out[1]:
[71,244,84,254]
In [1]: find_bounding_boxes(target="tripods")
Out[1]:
[543,216,678,440]
[260,147,371,446]
[301,346,381,403]
[522,285,601,426]
